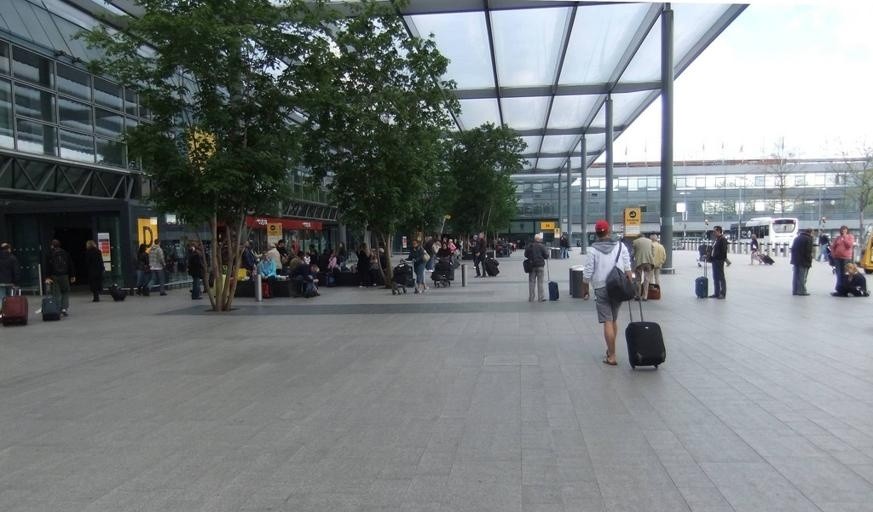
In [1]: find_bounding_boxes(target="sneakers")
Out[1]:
[708,293,718,298]
[716,293,726,300]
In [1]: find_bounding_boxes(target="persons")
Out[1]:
[400,231,515,294]
[790,224,868,297]
[559,234,570,259]
[524,233,550,302]
[241,239,387,299]
[43,238,75,317]
[578,219,634,365]
[83,239,214,302]
[0,243,20,319]
[700,226,760,299]
[631,232,666,302]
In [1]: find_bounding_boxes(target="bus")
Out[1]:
[729,216,799,253]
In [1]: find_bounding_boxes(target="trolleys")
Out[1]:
[430,255,454,288]
[389,259,418,295]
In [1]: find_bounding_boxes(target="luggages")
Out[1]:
[627,299,666,370]
[696,260,710,300]
[485,258,500,278]
[105,276,127,301]
[759,253,775,266]
[547,259,561,301]
[41,285,61,321]
[2,283,29,327]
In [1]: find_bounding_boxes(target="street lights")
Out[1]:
[816,188,827,237]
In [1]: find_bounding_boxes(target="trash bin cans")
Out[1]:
[551,248,560,259]
[569,265,583,298]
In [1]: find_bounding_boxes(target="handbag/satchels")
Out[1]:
[391,262,410,287]
[432,261,454,280]
[522,259,535,274]
[640,283,660,299]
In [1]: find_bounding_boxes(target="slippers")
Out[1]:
[601,349,619,367]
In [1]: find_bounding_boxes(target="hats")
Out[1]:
[593,219,611,232]
